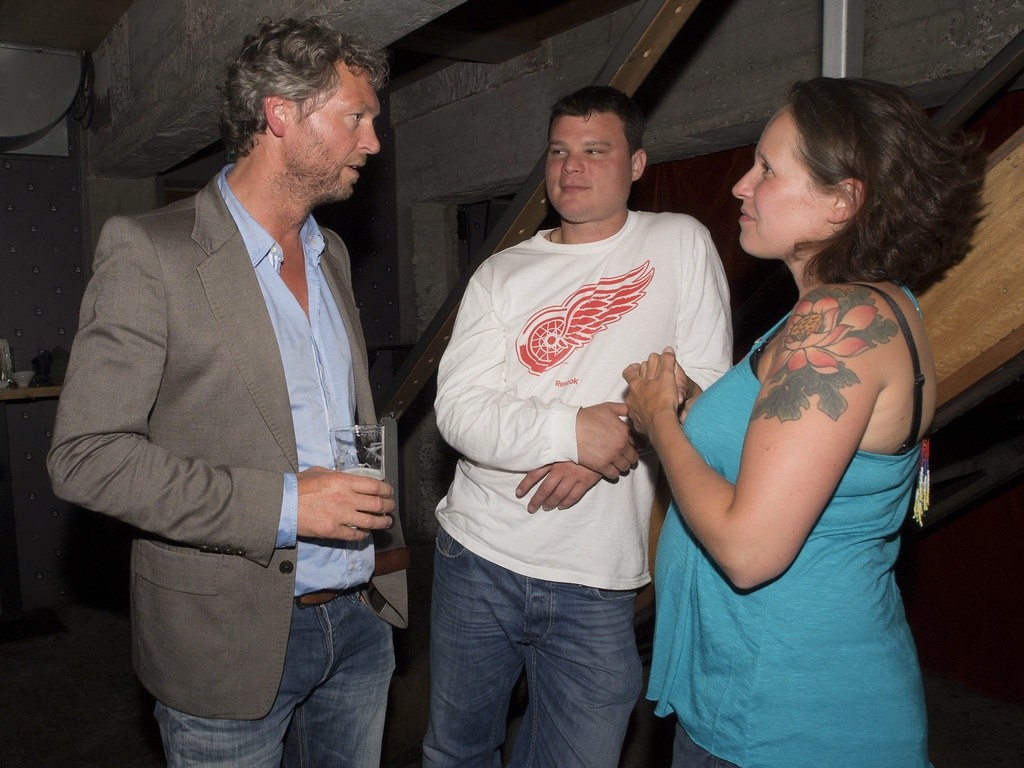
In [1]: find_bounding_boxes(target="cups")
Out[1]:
[0,339,11,388]
[329,423,386,532]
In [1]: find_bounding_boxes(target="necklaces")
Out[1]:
[550,228,558,242]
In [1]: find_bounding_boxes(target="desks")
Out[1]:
[0,385,70,644]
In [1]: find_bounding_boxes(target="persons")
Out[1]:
[621,76,985,768]
[44,15,409,768]
[422,86,732,768]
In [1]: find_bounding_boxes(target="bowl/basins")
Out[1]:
[12,371,35,386]
